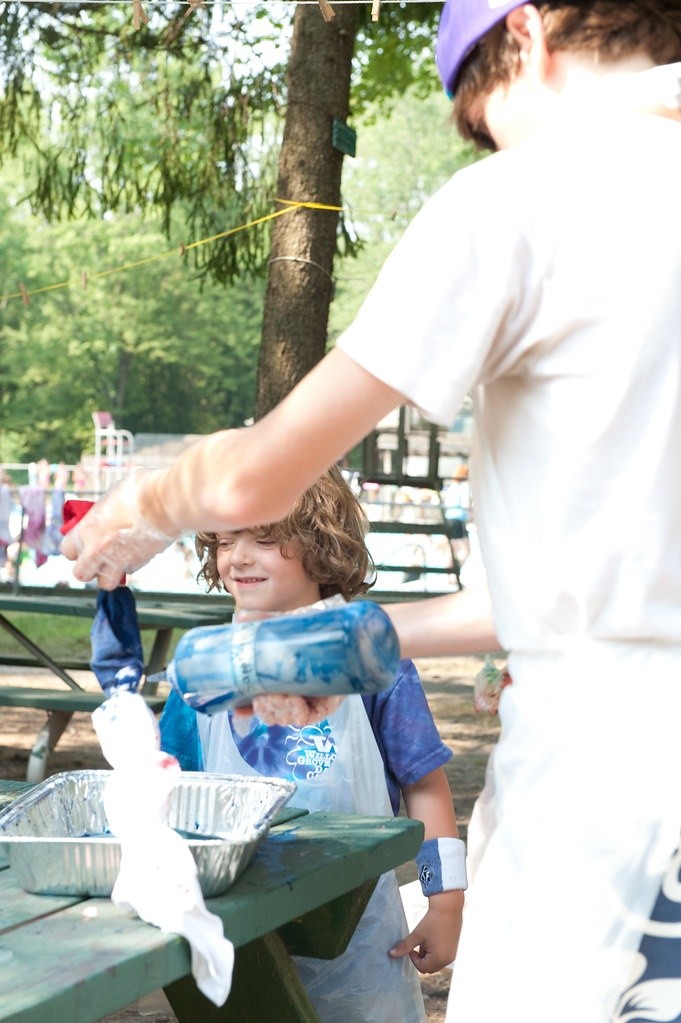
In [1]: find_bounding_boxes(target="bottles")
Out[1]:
[145,598,401,715]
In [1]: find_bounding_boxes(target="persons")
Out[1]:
[156,459,469,1021]
[56,0,681,1023]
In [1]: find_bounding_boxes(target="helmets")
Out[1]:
[436,1,529,102]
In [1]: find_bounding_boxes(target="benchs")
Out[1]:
[0,687,167,714]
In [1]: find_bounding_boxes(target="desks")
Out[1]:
[1,779,426,1023]
[1,592,235,782]
